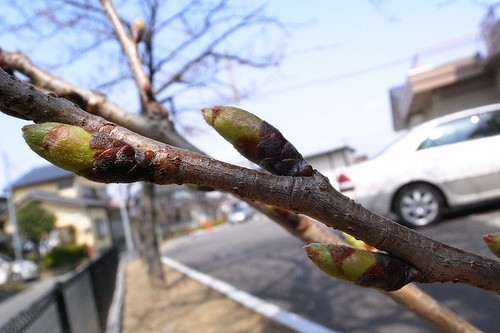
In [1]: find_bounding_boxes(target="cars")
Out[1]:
[335,100,499,228]
